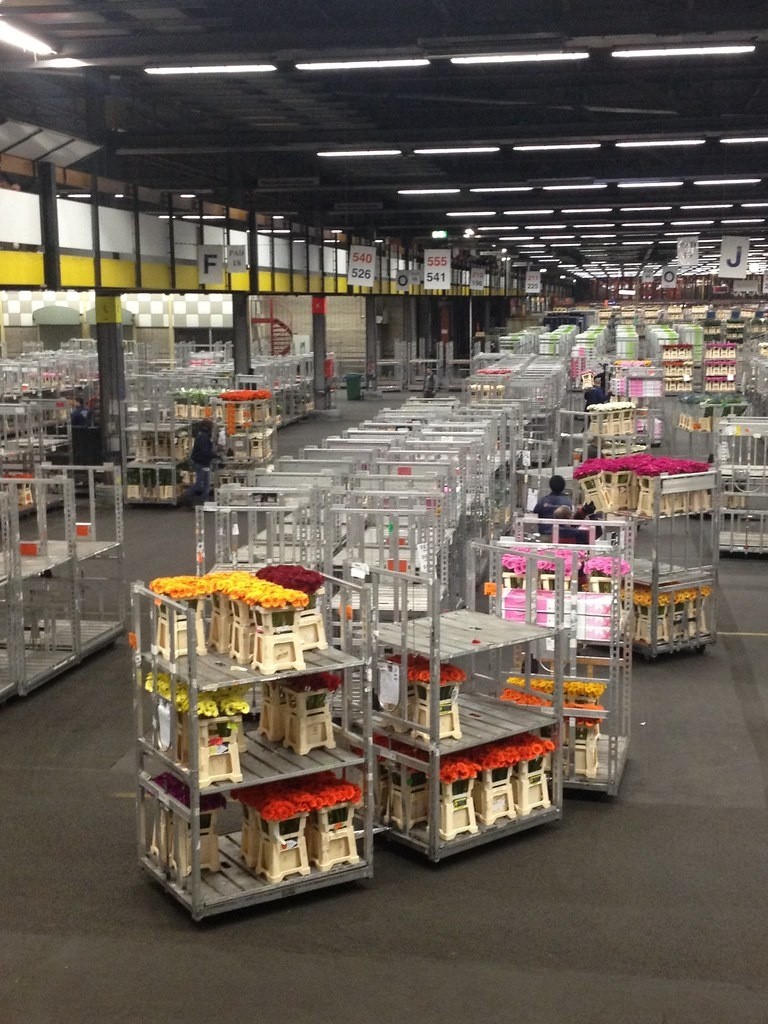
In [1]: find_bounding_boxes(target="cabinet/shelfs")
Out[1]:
[0,465,125,702]
[344,539,566,863]
[464,323,768,459]
[213,396,276,494]
[486,516,636,796]
[120,399,195,505]
[572,468,723,662]
[132,571,371,921]
[0,343,100,513]
[123,339,319,432]
[712,415,768,555]
[194,389,567,611]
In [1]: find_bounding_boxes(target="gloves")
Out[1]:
[582,501,596,515]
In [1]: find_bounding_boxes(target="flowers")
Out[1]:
[13,473,34,489]
[147,566,325,628]
[497,678,608,738]
[354,726,557,795]
[572,455,711,477]
[147,772,229,830]
[1,473,12,479]
[584,582,711,608]
[387,654,467,705]
[502,545,631,580]
[263,672,344,709]
[231,771,363,834]
[145,671,254,738]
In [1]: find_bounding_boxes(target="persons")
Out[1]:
[580,436,606,463]
[71,397,104,487]
[533,475,572,535]
[594,363,612,389]
[553,501,602,544]
[490,341,500,362]
[581,378,613,433]
[181,419,222,510]
[422,368,439,398]
